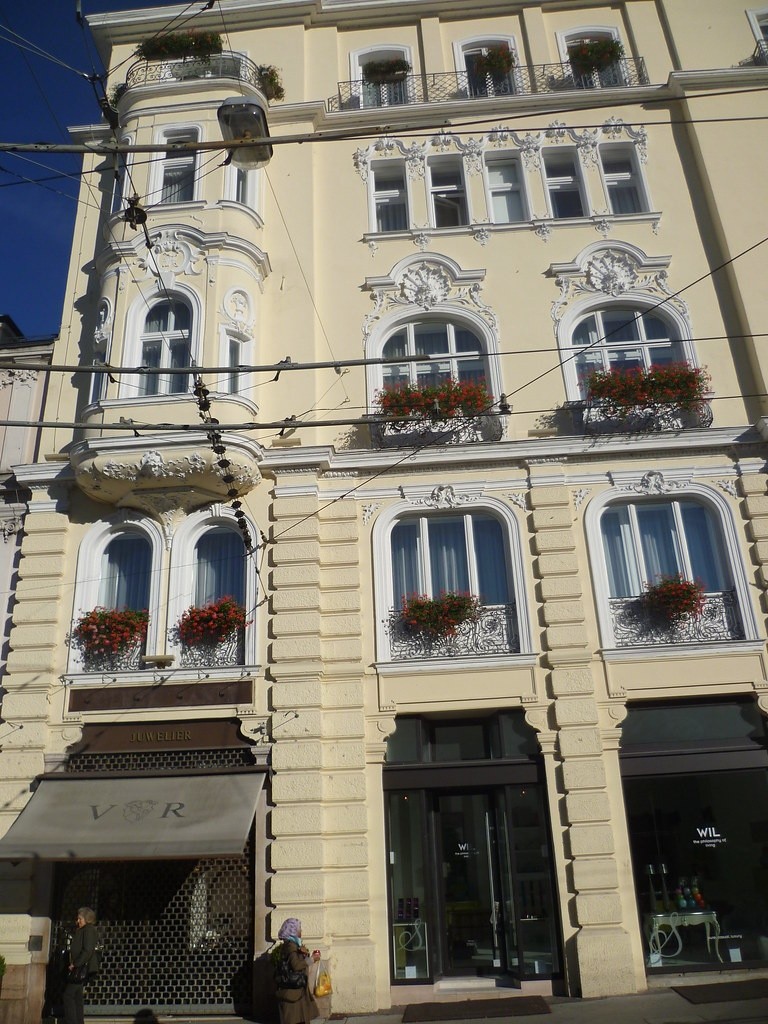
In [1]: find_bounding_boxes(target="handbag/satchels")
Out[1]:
[313,958,333,997]
[274,951,308,989]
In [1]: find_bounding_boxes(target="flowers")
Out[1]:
[635,573,706,623]
[469,41,517,79]
[392,588,483,639]
[67,603,152,660]
[579,361,714,405]
[371,377,495,417]
[260,65,285,101]
[565,39,625,72]
[138,29,223,67]
[175,593,254,645]
[360,56,412,81]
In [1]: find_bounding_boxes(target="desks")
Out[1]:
[652,909,725,963]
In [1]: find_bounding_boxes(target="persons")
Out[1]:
[273,918,323,1024]
[61,907,97,1024]
